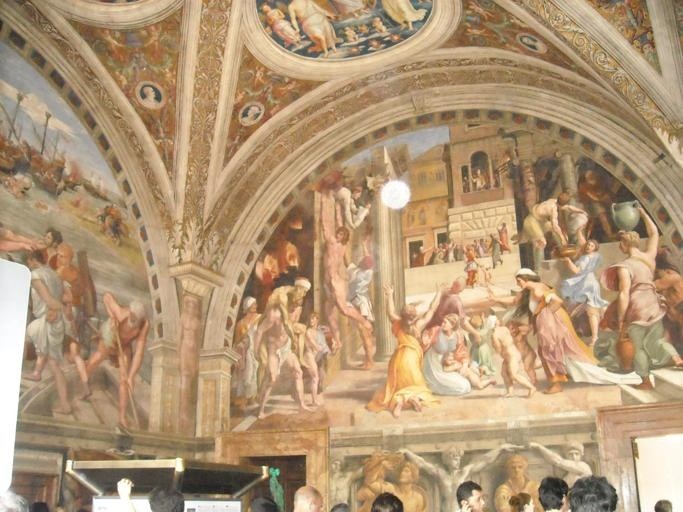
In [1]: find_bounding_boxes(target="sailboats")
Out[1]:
[0,92,82,198]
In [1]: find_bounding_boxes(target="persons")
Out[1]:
[371,491,402,511]
[397,442,524,512]
[260,2,428,57]
[1,228,150,437]
[654,499,672,512]
[457,477,619,512]
[247,497,278,512]
[1,490,29,512]
[318,453,366,512]
[330,502,352,512]
[117,477,185,512]
[293,486,323,511]
[179,295,202,418]
[529,438,593,486]
[385,463,426,512]
[493,454,543,512]
